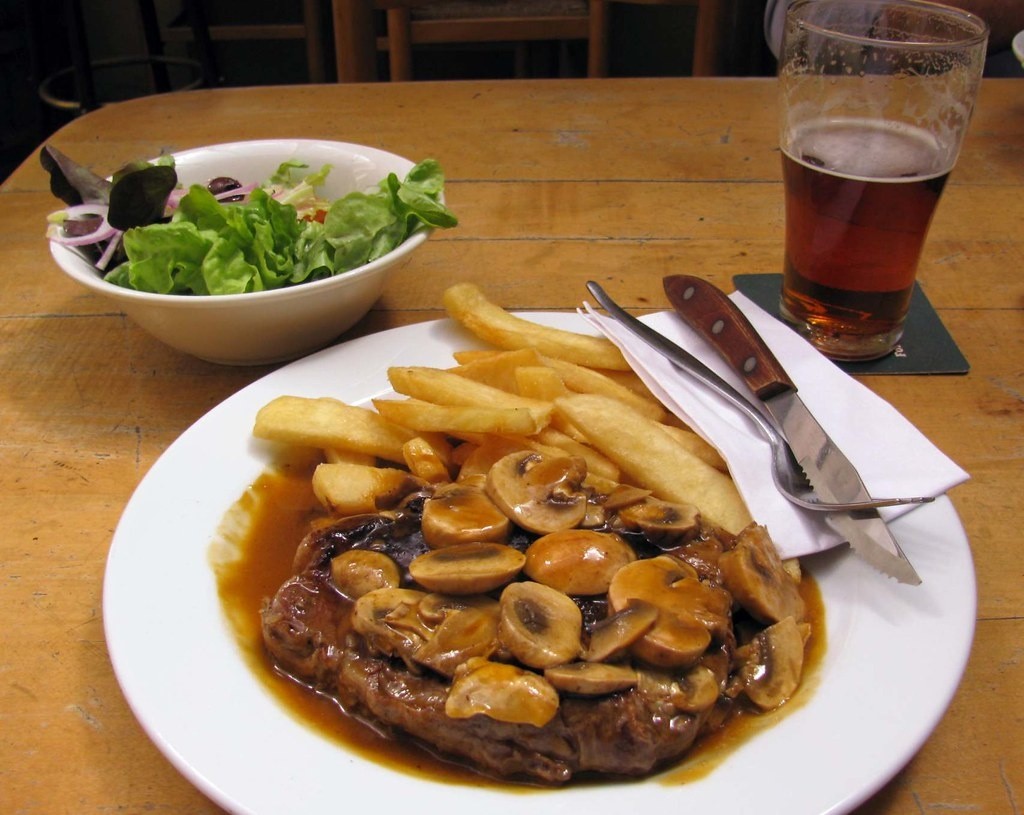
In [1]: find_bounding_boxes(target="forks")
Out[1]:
[587,278,939,512]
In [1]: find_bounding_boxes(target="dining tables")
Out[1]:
[0,68,1022,814]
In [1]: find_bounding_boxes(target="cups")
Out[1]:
[776,2,987,361]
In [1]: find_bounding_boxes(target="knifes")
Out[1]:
[661,272,923,589]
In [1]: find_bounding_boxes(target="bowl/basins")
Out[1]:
[48,133,441,370]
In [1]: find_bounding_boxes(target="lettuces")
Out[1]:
[38,145,459,293]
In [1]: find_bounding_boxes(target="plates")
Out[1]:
[101,310,981,815]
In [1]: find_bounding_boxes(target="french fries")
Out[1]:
[253,280,805,585]
